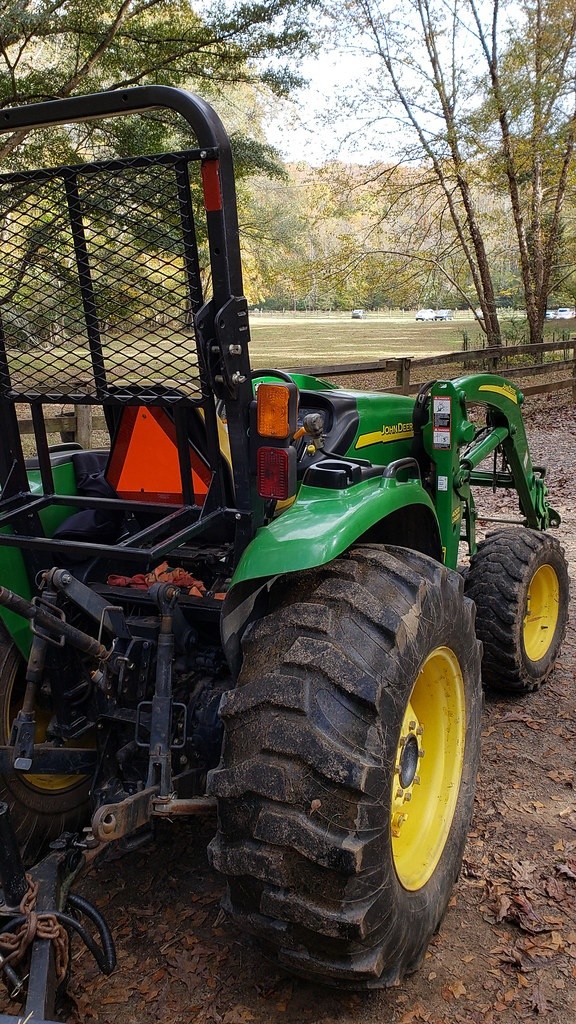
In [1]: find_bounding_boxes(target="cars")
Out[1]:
[557,308,576,320]
[415,309,436,321]
[351,310,367,319]
[474,308,498,320]
[435,310,454,321]
[546,312,555,319]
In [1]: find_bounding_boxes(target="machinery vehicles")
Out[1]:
[0,86,571,1024]
